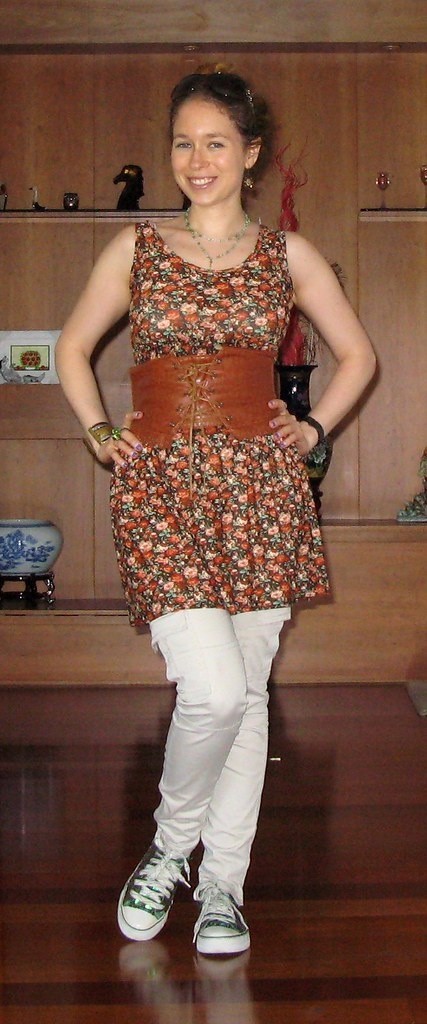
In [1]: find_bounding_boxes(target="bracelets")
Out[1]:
[82,422,113,456]
[301,416,325,446]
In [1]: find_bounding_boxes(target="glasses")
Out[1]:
[171,72,255,117]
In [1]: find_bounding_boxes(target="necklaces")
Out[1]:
[184,205,252,269]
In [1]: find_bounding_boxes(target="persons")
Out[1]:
[52,65,380,957]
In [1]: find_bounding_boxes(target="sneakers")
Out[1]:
[118,838,191,940]
[193,881,250,953]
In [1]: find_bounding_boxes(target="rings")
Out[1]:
[112,426,121,441]
